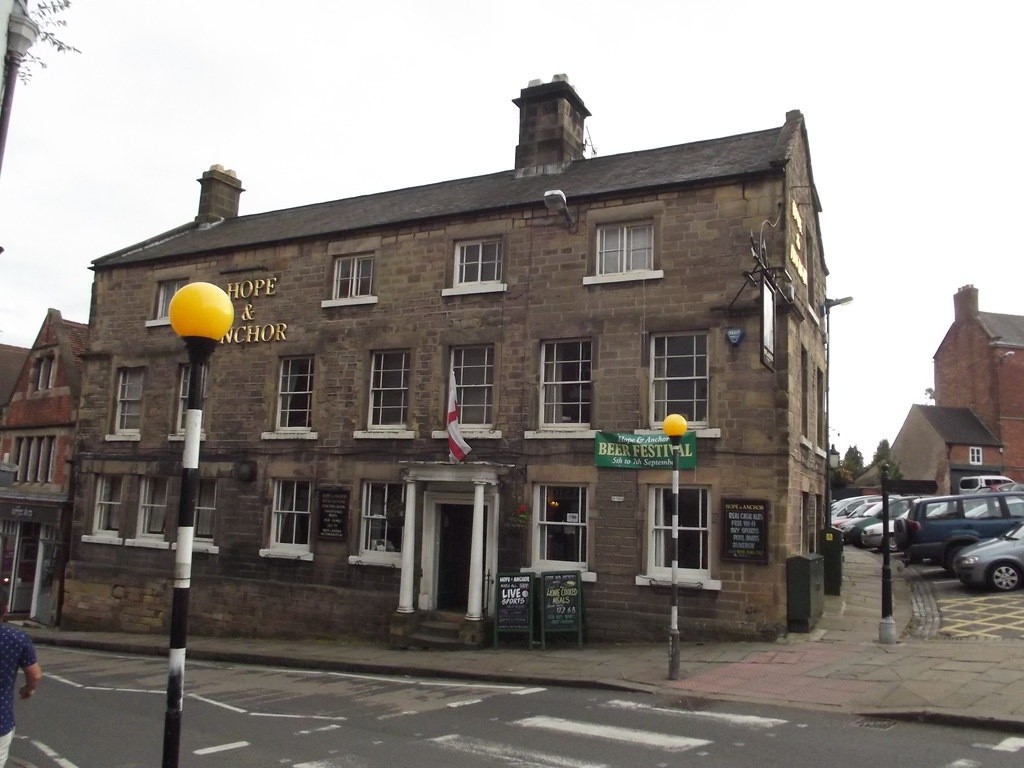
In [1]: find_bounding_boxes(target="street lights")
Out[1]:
[160,281,234,768]
[663,413,688,680]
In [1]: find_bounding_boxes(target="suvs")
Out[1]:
[893,491,1024,571]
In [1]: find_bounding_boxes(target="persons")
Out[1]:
[0,585,42,768]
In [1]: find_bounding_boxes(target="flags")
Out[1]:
[447,374,472,462]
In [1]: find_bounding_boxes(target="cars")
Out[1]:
[953,522,1024,591]
[830,482,1024,552]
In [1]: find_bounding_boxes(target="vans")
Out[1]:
[959,475,1015,496]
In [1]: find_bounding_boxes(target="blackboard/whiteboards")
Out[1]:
[316,488,350,541]
[495,572,534,634]
[540,569,583,633]
[721,498,769,564]
